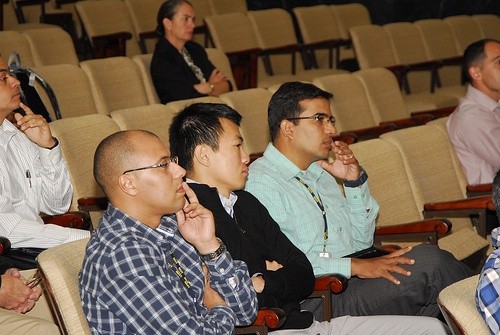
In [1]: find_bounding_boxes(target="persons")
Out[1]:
[475,174,499,333]
[0,56,91,247]
[168,102,450,333]
[448,38,499,184]
[78,129,260,335]
[150,1,234,105]
[1,268,62,335]
[244,81,479,323]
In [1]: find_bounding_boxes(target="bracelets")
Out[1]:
[207,80,216,94]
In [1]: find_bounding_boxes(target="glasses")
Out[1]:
[287,113,336,125]
[26,274,42,289]
[123,156,178,174]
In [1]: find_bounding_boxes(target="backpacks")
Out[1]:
[5,51,62,123]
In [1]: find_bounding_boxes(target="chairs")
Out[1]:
[0,0,500,335]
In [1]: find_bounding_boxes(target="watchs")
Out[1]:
[343,170,368,188]
[199,237,226,262]
